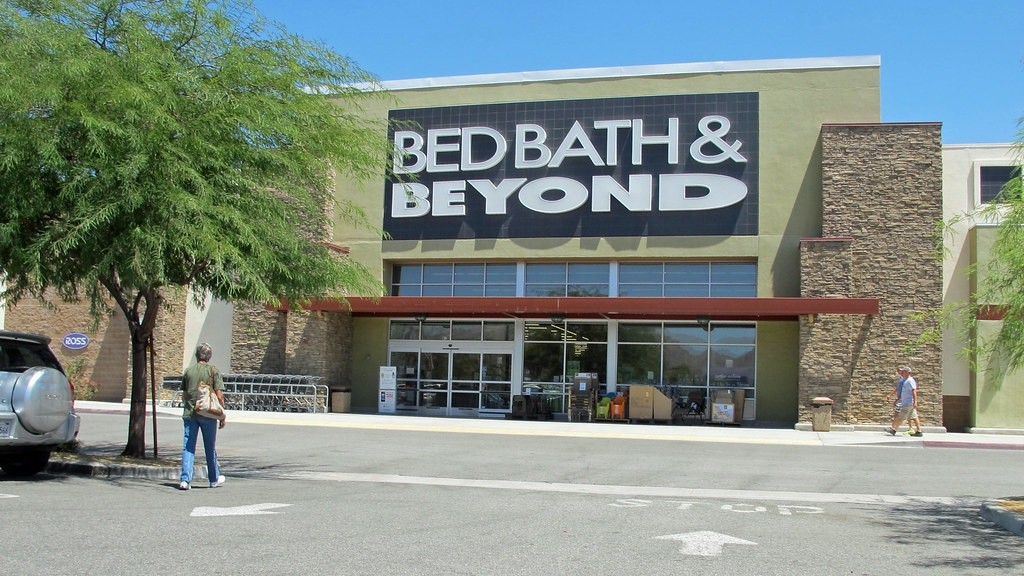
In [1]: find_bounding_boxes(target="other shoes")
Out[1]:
[910,431,923,437]
[903,428,915,435]
[884,427,896,434]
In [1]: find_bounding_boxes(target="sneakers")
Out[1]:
[210,475,225,487]
[180,481,191,490]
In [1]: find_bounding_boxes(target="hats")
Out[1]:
[901,367,911,372]
[895,365,905,370]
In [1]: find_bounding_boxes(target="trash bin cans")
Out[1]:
[330,386,351,412]
[811,396,834,432]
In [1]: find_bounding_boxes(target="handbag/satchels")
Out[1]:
[195,365,227,420]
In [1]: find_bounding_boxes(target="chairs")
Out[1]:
[671,391,704,426]
[611,396,627,419]
[596,397,611,419]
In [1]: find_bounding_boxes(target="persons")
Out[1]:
[883,365,922,437]
[180,343,225,489]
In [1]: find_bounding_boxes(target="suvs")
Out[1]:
[0,330,81,477]
